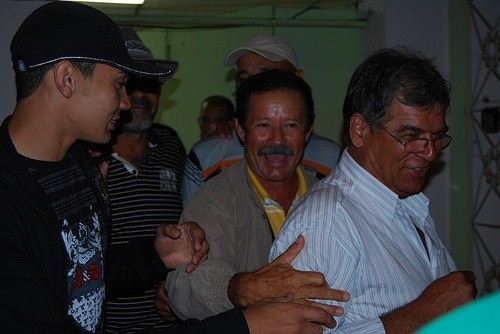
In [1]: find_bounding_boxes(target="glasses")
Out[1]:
[374,123,453,154]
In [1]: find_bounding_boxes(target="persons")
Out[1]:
[184,34,343,179]
[165,67,351,324]
[196,94,236,139]
[89,26,204,334]
[269,48,477,334]
[1,1,340,334]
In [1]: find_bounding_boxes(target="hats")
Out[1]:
[117,28,179,83]
[10,0,173,77]
[223,34,301,71]
